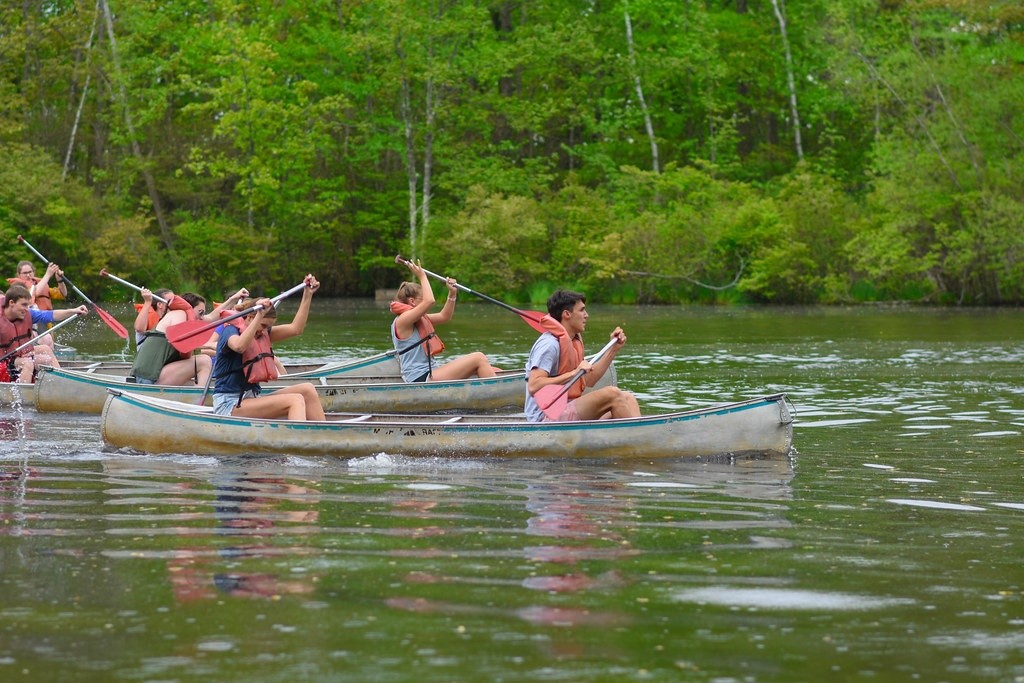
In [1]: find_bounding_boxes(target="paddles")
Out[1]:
[99,267,166,312]
[393,252,549,336]
[164,279,312,353]
[16,233,128,340]
[270,290,287,316]
[0,312,77,363]
[235,292,244,311]
[533,335,620,419]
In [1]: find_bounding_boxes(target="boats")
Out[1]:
[96,383,799,473]
[32,361,537,414]
[0,350,404,389]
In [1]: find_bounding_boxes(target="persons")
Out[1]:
[0,260,89,384]
[524,288,641,423]
[135,286,175,352]
[5,260,68,339]
[212,273,326,421]
[390,258,498,383]
[130,287,250,387]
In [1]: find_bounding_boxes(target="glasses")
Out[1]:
[20,271,33,274]
[260,322,271,331]
[193,308,205,317]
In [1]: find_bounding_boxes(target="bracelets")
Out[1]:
[447,297,456,301]
[58,279,65,283]
